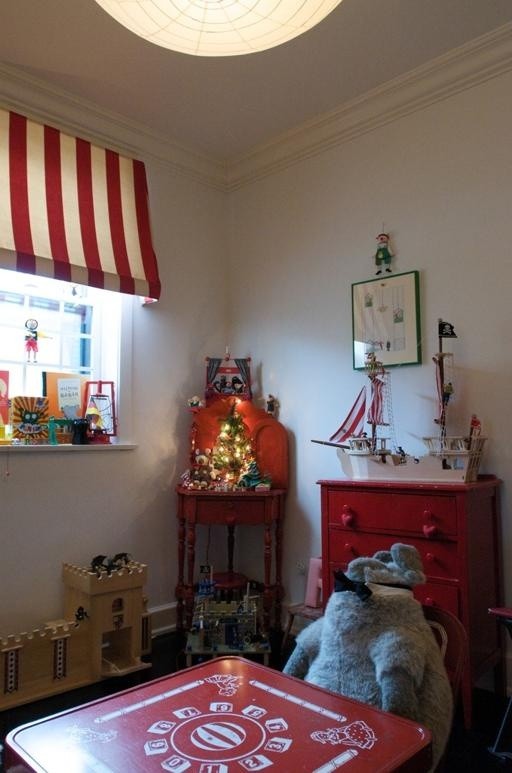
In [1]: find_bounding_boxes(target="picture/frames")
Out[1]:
[351,270,422,370]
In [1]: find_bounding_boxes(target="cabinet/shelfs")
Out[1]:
[316,476,508,731]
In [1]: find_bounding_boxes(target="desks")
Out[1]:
[5,655,435,773]
[174,485,289,647]
